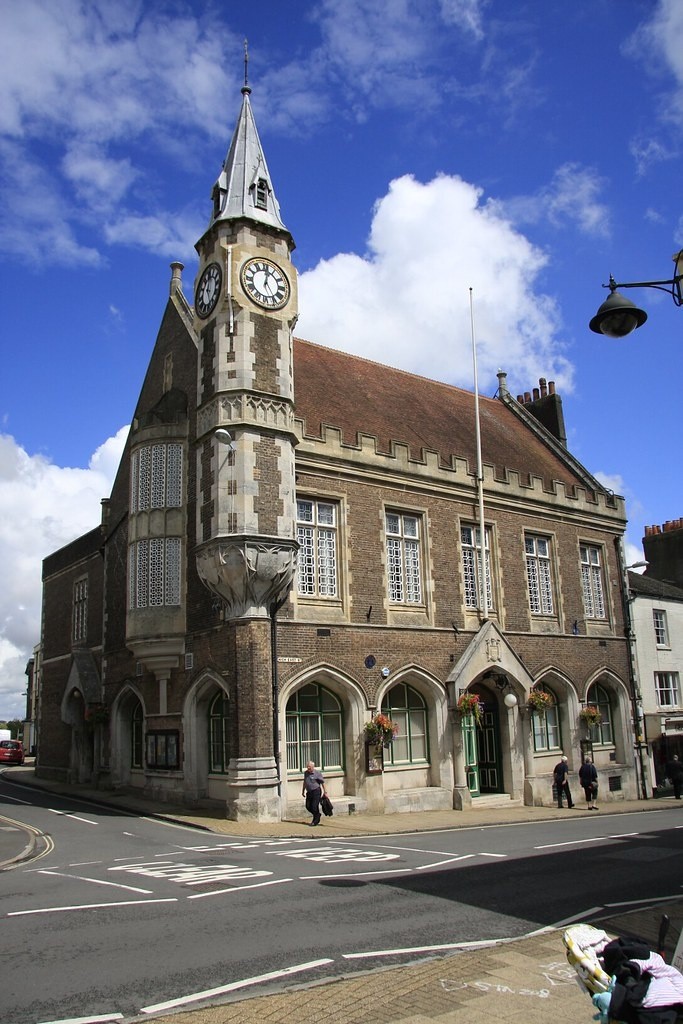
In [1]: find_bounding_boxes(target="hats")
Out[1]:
[562,756,567,761]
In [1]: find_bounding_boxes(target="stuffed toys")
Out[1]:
[592,975,617,1024]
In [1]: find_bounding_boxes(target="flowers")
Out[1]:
[578,707,604,729]
[527,688,552,711]
[457,693,486,722]
[364,713,399,749]
[84,705,109,722]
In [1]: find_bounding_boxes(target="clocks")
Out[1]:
[193,263,223,319]
[240,256,291,310]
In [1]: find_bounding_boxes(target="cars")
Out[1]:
[0,739,28,766]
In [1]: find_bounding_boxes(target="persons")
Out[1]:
[553,755,575,808]
[579,757,599,810]
[300,760,328,826]
[666,755,683,799]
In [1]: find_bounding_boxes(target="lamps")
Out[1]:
[73,689,80,697]
[485,673,517,707]
[214,428,234,449]
[625,560,650,570]
[381,668,389,676]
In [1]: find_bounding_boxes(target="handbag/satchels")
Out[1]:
[590,781,598,791]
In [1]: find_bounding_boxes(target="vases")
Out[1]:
[580,715,595,721]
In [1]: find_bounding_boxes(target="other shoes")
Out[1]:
[558,806,564,808]
[592,806,599,810]
[568,804,575,808]
[311,816,320,826]
[588,807,592,810]
[676,797,681,799]
